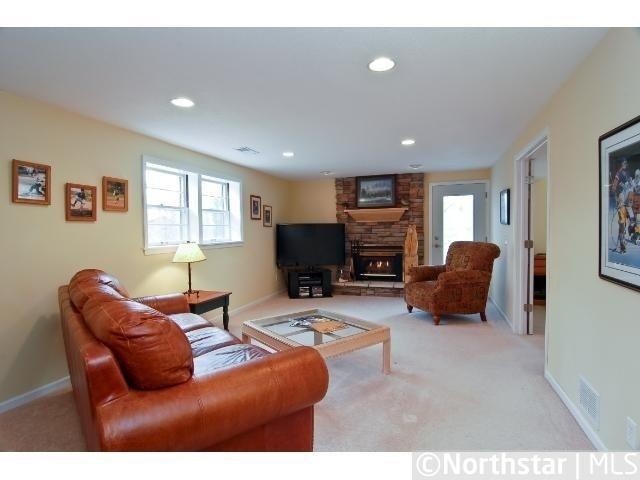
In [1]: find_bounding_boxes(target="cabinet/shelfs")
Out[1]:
[285,268,334,299]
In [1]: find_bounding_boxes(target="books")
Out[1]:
[299,287,309,296]
[313,286,323,296]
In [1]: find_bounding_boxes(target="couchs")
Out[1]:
[57,268,329,451]
[400,238,501,326]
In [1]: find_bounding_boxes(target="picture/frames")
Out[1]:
[10,157,52,208]
[595,115,640,295]
[250,194,261,220]
[100,175,130,214]
[263,204,273,227]
[63,181,98,223]
[499,188,510,225]
[354,173,399,209]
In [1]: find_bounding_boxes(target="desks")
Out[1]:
[180,289,231,335]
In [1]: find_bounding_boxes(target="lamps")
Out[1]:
[171,239,209,299]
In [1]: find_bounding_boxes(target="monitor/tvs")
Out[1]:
[276,223,345,271]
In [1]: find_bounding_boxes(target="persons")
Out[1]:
[24,170,46,196]
[71,187,86,208]
[612,157,633,254]
[112,184,121,196]
